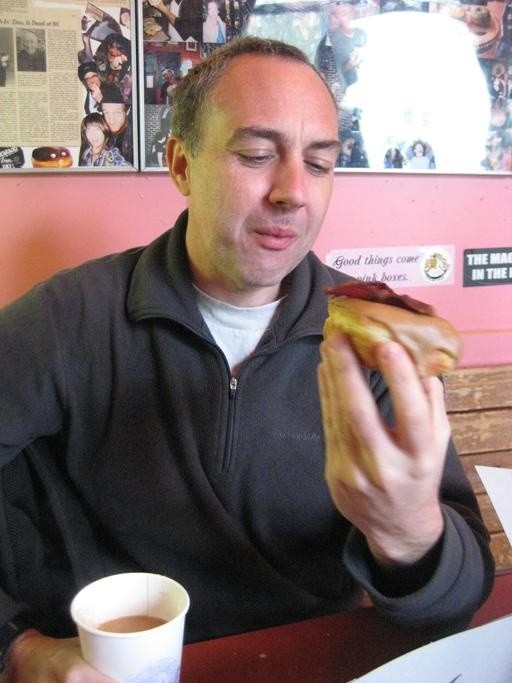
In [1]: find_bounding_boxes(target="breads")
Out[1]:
[323,281,461,378]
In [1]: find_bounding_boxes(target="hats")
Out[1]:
[102,87,126,104]
[77,63,100,80]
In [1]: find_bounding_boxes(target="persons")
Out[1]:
[246,0,511,177]
[0,38,494,682]
[80,0,252,169]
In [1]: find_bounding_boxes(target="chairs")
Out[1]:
[436,363,512,576]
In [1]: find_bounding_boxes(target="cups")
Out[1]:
[69,569,192,681]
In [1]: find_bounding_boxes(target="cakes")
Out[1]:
[448,5,466,20]
[469,6,491,26]
